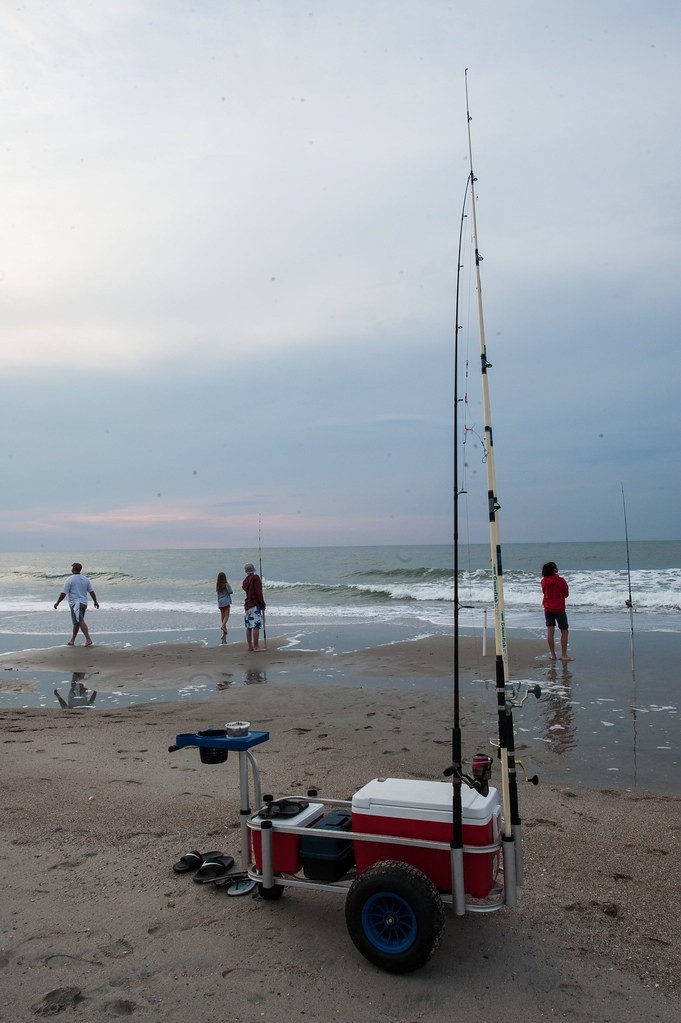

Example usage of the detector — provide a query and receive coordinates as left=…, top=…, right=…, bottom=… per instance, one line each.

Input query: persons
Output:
left=53, top=672, right=97, bottom=709
left=241, top=563, right=268, bottom=652
left=215, top=572, right=233, bottom=633
left=54, top=563, right=99, bottom=647
left=540, top=562, right=576, bottom=661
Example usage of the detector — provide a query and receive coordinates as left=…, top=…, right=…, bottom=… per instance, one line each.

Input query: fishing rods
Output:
left=258, top=513, right=269, bottom=649
left=442, top=174, right=475, bottom=914
left=463, top=66, right=526, bottom=886
left=627, top=610, right=638, bottom=784
left=620, top=480, right=633, bottom=609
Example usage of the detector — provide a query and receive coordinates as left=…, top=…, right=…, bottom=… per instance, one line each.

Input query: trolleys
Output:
left=164, top=725, right=520, bottom=972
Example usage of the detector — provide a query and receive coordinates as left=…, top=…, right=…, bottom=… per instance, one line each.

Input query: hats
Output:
left=243, top=564, right=255, bottom=574
left=72, top=562, right=83, bottom=571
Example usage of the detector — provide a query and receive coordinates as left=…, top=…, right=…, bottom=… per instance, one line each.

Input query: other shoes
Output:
left=560, top=656, right=574, bottom=661
left=549, top=655, right=556, bottom=660
